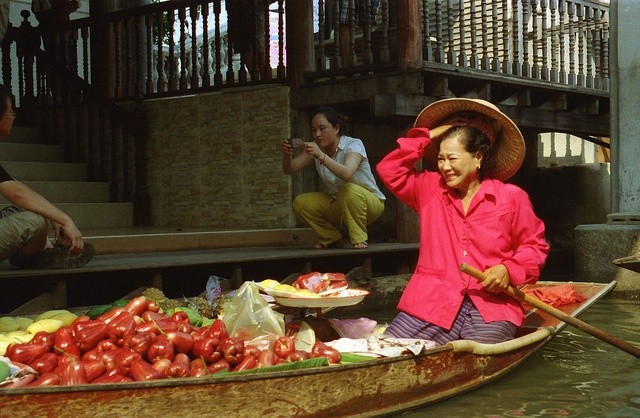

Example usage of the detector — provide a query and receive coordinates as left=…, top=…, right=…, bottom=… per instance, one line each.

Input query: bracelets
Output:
left=319, top=153, right=327, bottom=165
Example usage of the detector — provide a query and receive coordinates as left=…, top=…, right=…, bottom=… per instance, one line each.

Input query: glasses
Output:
left=2, top=111, right=16, bottom=119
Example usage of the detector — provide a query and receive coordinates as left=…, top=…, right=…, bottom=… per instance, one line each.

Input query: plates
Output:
left=264, top=286, right=372, bottom=307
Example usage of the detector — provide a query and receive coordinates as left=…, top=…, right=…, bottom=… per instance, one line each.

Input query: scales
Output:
left=273, top=288, right=370, bottom=344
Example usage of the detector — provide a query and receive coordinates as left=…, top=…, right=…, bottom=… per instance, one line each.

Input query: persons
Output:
left=281, top=105, right=386, bottom=249
left=1, top=84, right=85, bottom=264
left=376, top=98, right=552, bottom=347
left=331, top=1, right=379, bottom=70
left=225, top=0, right=276, bottom=81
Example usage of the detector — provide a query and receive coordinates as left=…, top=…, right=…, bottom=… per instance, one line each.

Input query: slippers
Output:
left=353, top=240, right=368, bottom=249
left=313, top=243, right=332, bottom=249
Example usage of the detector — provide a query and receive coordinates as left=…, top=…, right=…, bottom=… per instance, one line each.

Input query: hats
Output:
left=413, top=98, right=526, bottom=182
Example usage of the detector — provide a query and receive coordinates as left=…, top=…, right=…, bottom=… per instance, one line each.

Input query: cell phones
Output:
left=287, top=138, right=303, bottom=148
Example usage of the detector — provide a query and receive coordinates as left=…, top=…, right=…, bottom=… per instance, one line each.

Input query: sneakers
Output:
left=28, top=242, right=95, bottom=268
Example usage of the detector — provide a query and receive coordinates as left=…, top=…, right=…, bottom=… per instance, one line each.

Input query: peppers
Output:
left=292, top=271, right=350, bottom=296
left=6, top=295, right=342, bottom=389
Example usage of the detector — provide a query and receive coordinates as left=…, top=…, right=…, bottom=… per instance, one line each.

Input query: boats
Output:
left=0, top=272, right=616, bottom=418
